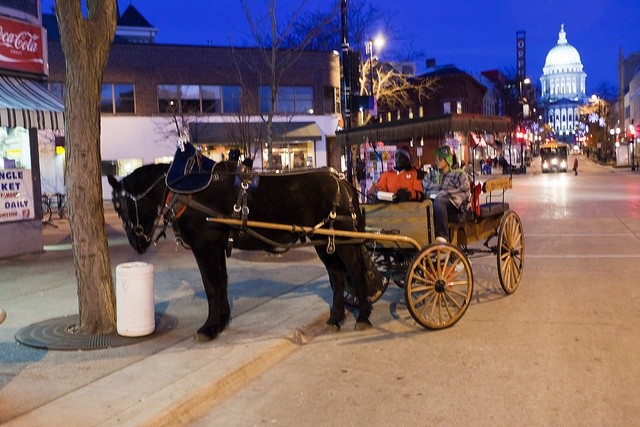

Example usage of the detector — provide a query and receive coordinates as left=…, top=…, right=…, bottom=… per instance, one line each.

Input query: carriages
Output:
left=106, top=115, right=524, bottom=343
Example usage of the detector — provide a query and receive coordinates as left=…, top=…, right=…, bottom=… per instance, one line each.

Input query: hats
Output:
left=395, top=146, right=411, bottom=161
left=435, top=146, right=453, bottom=165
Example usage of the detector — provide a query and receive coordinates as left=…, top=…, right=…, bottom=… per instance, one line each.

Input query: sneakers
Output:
left=436, top=236, right=448, bottom=244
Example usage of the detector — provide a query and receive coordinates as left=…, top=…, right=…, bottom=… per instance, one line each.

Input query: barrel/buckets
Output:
left=114, top=260, right=156, bottom=338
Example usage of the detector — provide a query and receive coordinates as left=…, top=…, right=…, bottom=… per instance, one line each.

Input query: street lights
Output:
left=629, top=118, right=637, bottom=171
left=609, top=127, right=621, bottom=145
left=518, top=77, right=532, bottom=115
left=367, top=32, right=385, bottom=99
left=592, top=94, right=601, bottom=120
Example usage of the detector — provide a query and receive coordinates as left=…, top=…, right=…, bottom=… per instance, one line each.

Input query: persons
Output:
left=461, top=159, right=466, bottom=176
left=369, top=145, right=423, bottom=204
left=298, top=152, right=304, bottom=168
left=295, top=155, right=299, bottom=169
left=308, top=160, right=313, bottom=169
left=452, top=149, right=459, bottom=169
left=355, top=154, right=367, bottom=205
left=480, top=160, right=490, bottom=174
left=470, top=173, right=481, bottom=223
left=487, top=156, right=492, bottom=168
left=573, top=157, right=579, bottom=174
left=501, top=157, right=508, bottom=170
left=499, top=153, right=503, bottom=163
left=468, top=162, right=473, bottom=177
left=243, top=158, right=253, bottom=170
left=284, top=164, right=288, bottom=173
left=228, top=149, right=240, bottom=162
left=421, top=144, right=471, bottom=245
left=494, top=156, right=498, bottom=167
left=270, top=156, right=282, bottom=170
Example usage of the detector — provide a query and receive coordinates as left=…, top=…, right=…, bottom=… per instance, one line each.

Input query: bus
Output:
left=539, top=140, right=570, bottom=172
left=501, top=143, right=527, bottom=175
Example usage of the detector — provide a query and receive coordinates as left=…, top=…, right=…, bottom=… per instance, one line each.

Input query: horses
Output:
left=105, top=160, right=376, bottom=345
